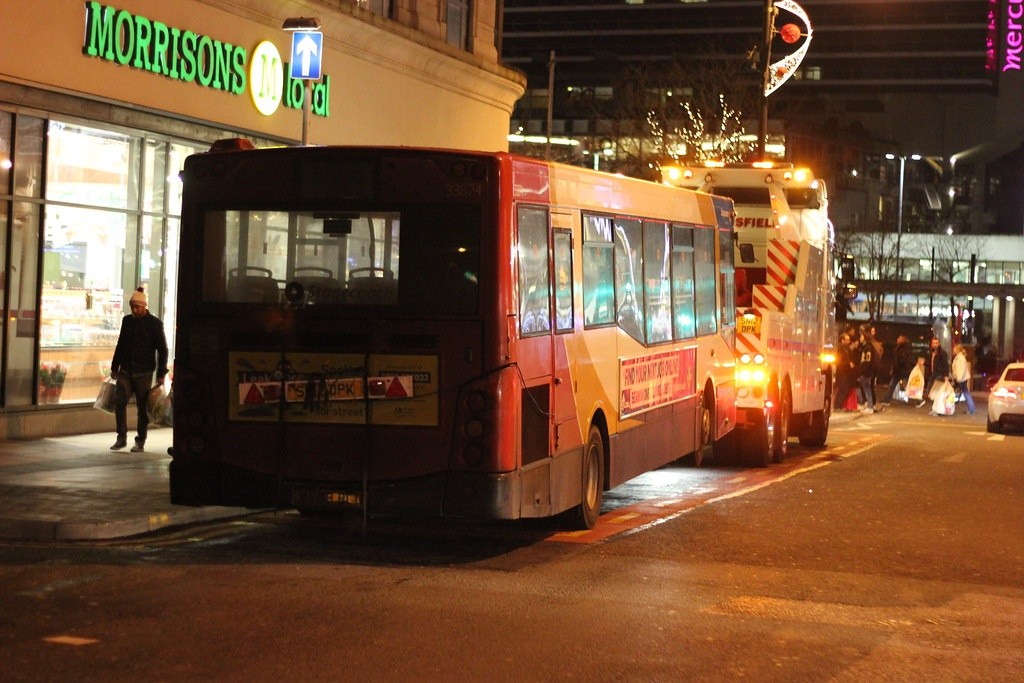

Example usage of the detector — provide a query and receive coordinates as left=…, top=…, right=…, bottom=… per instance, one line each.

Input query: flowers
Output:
left=40, top=363, right=67, bottom=389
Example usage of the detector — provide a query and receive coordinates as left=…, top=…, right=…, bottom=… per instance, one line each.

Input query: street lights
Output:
left=885, top=153, right=921, bottom=323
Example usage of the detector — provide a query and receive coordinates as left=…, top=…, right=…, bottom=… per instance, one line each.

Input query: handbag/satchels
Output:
left=93, top=380, right=116, bottom=416
left=147, top=383, right=174, bottom=427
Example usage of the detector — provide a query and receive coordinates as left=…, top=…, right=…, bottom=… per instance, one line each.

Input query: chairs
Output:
left=284, top=266, right=344, bottom=304
left=226, top=266, right=278, bottom=304
left=348, top=267, right=398, bottom=305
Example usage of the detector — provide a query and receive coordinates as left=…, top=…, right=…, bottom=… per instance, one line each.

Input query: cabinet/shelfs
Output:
left=39, top=287, right=125, bottom=382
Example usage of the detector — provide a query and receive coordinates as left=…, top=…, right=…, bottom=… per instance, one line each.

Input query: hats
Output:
left=129, top=286, right=149, bottom=307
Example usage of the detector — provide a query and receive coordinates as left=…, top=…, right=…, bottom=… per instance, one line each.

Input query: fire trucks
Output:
left=654, top=161, right=835, bottom=469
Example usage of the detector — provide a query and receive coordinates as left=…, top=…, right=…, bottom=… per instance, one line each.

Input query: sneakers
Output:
left=131, top=445, right=144, bottom=453
left=109, top=440, right=126, bottom=450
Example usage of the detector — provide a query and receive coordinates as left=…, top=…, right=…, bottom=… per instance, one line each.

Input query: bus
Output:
left=167, top=138, right=737, bottom=529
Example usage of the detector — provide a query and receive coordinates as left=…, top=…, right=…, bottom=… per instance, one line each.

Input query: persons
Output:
left=838, top=324, right=975, bottom=414
left=110, top=286, right=169, bottom=452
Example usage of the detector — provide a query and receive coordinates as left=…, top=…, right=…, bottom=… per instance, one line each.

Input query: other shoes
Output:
left=880, top=401, right=891, bottom=407
left=963, top=410, right=975, bottom=415
left=916, top=400, right=927, bottom=407
left=862, top=407, right=875, bottom=415
left=929, top=411, right=939, bottom=417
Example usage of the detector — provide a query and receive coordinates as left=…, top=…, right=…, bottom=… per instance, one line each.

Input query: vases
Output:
left=40, top=384, right=62, bottom=402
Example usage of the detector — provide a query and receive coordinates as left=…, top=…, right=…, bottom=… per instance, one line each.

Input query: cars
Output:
left=988, top=362, right=1024, bottom=432
